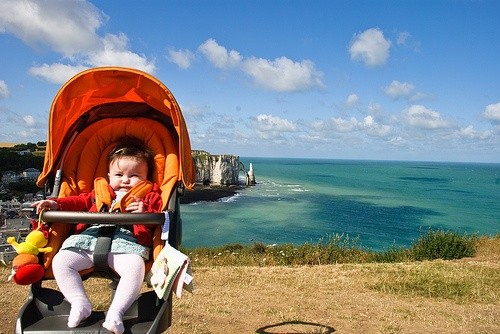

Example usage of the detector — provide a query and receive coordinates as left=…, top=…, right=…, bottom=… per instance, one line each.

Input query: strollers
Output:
left=15, top=67, right=196, bottom=333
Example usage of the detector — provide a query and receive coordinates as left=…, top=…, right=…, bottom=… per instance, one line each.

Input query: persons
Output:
left=31, top=143, right=163, bottom=334
